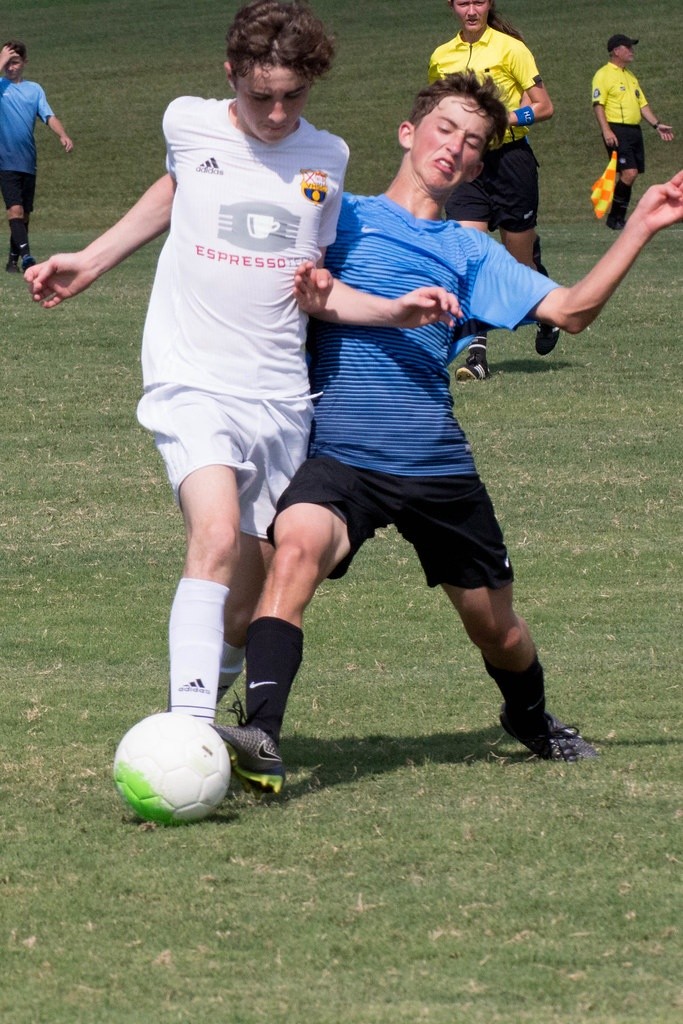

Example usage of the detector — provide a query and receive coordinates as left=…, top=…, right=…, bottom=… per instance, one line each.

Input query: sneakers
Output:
left=208, top=691, right=286, bottom=793
left=534, top=320, right=560, bottom=356
left=454, top=355, right=490, bottom=381
left=499, top=700, right=600, bottom=763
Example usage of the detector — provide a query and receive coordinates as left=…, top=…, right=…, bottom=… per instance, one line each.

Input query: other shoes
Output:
left=22, top=256, right=37, bottom=270
left=606, top=213, right=627, bottom=229
left=5, top=259, right=21, bottom=273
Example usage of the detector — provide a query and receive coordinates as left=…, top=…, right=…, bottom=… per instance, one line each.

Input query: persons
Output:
left=21, top=0, right=465, bottom=762
left=591, top=34, right=675, bottom=233
left=196, top=73, right=683, bottom=794
left=425, top=0, right=562, bottom=385
left=0, top=39, right=74, bottom=274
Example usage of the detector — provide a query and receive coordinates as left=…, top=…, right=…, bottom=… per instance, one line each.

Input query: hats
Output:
left=606, top=33, right=640, bottom=54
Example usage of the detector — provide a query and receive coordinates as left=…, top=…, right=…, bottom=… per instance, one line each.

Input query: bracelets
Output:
left=652, top=120, right=661, bottom=129
left=513, top=106, right=535, bottom=127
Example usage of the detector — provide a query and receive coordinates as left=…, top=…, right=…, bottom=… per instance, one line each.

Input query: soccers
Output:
left=106, top=708, right=239, bottom=830
